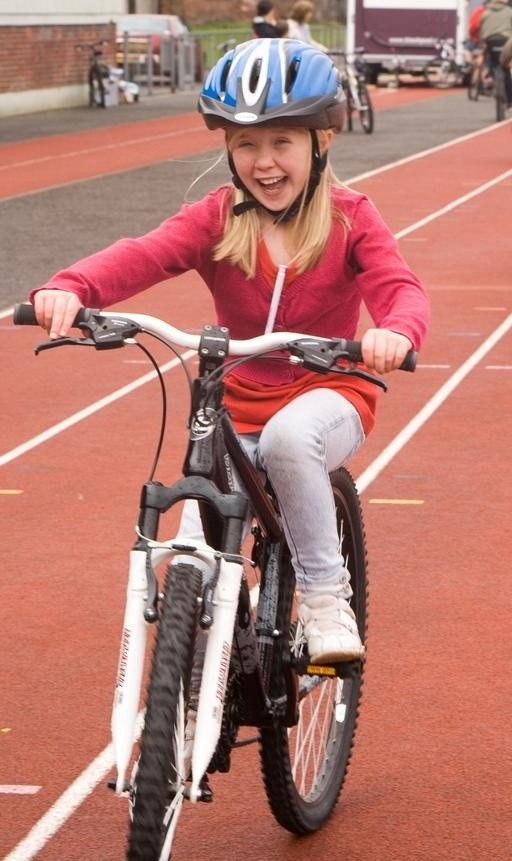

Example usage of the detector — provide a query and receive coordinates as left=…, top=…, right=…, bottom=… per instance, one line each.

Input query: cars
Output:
left=115, top=13, right=203, bottom=82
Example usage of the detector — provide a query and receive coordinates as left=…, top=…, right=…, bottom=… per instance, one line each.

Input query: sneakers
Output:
left=295, top=595, right=367, bottom=665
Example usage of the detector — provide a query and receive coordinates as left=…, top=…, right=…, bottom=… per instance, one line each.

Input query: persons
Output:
left=276, top=21, right=290, bottom=37
left=285, top=1, right=334, bottom=53
left=28, top=37, right=427, bottom=779
left=250, top=0, right=278, bottom=39
left=478, top=0, right=512, bottom=109
left=467, top=0, right=492, bottom=77
left=116, top=78, right=141, bottom=105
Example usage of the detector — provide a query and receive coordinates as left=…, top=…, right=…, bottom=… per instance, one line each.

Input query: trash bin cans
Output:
left=93, top=68, right=124, bottom=108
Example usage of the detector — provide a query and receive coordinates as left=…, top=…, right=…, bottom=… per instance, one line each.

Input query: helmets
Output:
left=197, top=37, right=347, bottom=133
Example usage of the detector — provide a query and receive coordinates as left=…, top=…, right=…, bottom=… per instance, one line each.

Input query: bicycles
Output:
left=334, top=47, right=374, bottom=136
left=13, top=304, right=418, bottom=861
left=424, top=44, right=511, bottom=122
left=72, top=39, right=112, bottom=109
